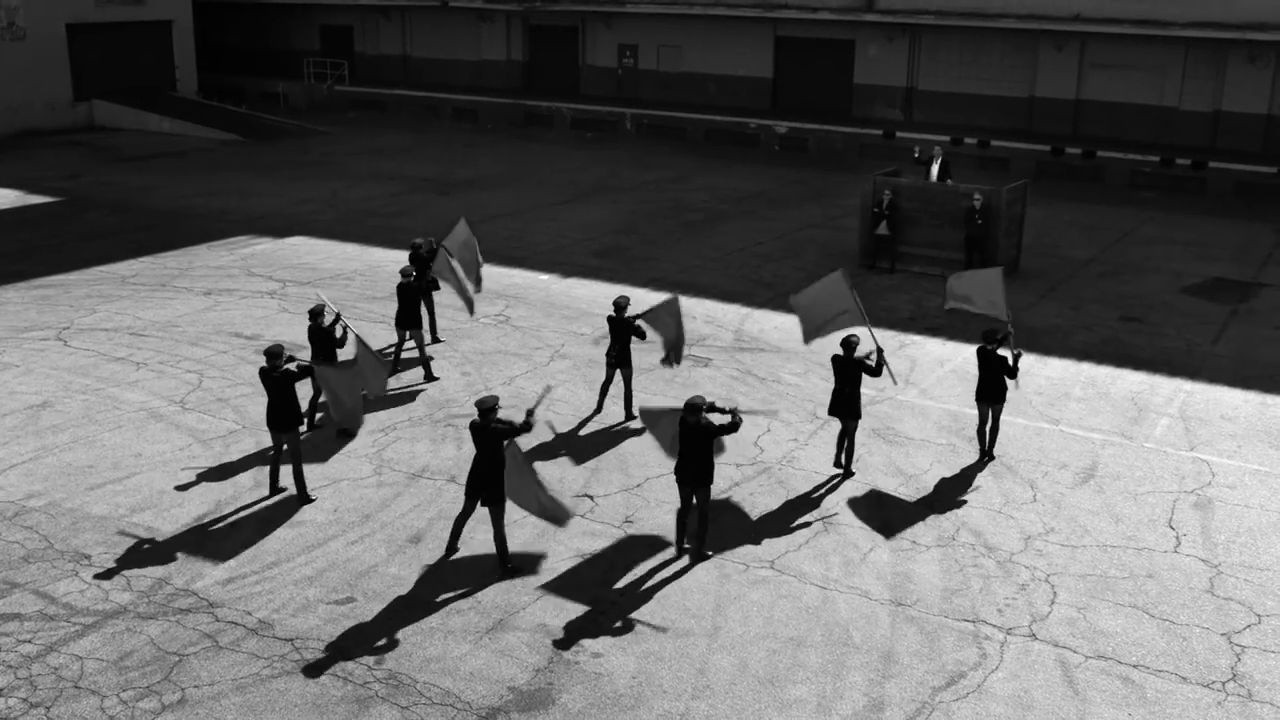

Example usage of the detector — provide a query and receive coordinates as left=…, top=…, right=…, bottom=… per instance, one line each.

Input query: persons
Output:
left=674, top=395, right=743, bottom=565
left=864, top=187, right=903, bottom=273
left=913, top=145, right=952, bottom=184
left=445, top=395, right=525, bottom=578
left=258, top=344, right=318, bottom=504
left=593, top=295, right=639, bottom=421
left=827, top=334, right=885, bottom=478
left=975, top=328, right=1023, bottom=462
left=392, top=237, right=446, bottom=381
left=960, top=190, right=992, bottom=270
left=306, top=304, right=357, bottom=437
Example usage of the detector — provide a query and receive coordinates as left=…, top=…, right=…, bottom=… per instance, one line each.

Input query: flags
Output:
left=639, top=296, right=685, bottom=368
left=312, top=335, right=392, bottom=427
left=504, top=439, right=571, bottom=527
left=944, top=267, right=1009, bottom=322
left=429, top=246, right=474, bottom=318
left=639, top=407, right=726, bottom=460
left=441, top=217, right=483, bottom=293
left=789, top=267, right=867, bottom=344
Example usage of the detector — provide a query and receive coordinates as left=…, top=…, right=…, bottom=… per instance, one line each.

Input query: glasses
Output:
left=972, top=198, right=981, bottom=201
left=881, top=193, right=891, bottom=196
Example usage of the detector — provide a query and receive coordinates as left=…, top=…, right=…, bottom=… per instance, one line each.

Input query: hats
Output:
left=399, top=265, right=415, bottom=276
left=982, top=328, right=1000, bottom=343
left=411, top=239, right=423, bottom=249
left=307, top=304, right=330, bottom=317
left=839, top=334, right=860, bottom=348
left=613, top=295, right=629, bottom=307
left=263, top=343, right=286, bottom=361
left=684, top=395, right=708, bottom=415
left=475, top=395, right=503, bottom=412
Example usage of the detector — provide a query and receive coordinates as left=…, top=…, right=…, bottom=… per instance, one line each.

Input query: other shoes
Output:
left=424, top=374, right=439, bottom=380
left=986, top=451, right=995, bottom=459
left=307, top=424, right=321, bottom=432
left=392, top=366, right=401, bottom=371
left=298, top=494, right=317, bottom=502
left=625, top=414, right=639, bottom=421
left=691, top=551, right=712, bottom=562
left=979, top=449, right=987, bottom=457
left=676, top=543, right=692, bottom=554
left=447, top=545, right=461, bottom=554
left=270, top=486, right=287, bottom=495
left=431, top=336, right=446, bottom=344
left=833, top=463, right=844, bottom=469
left=845, top=470, right=857, bottom=477
